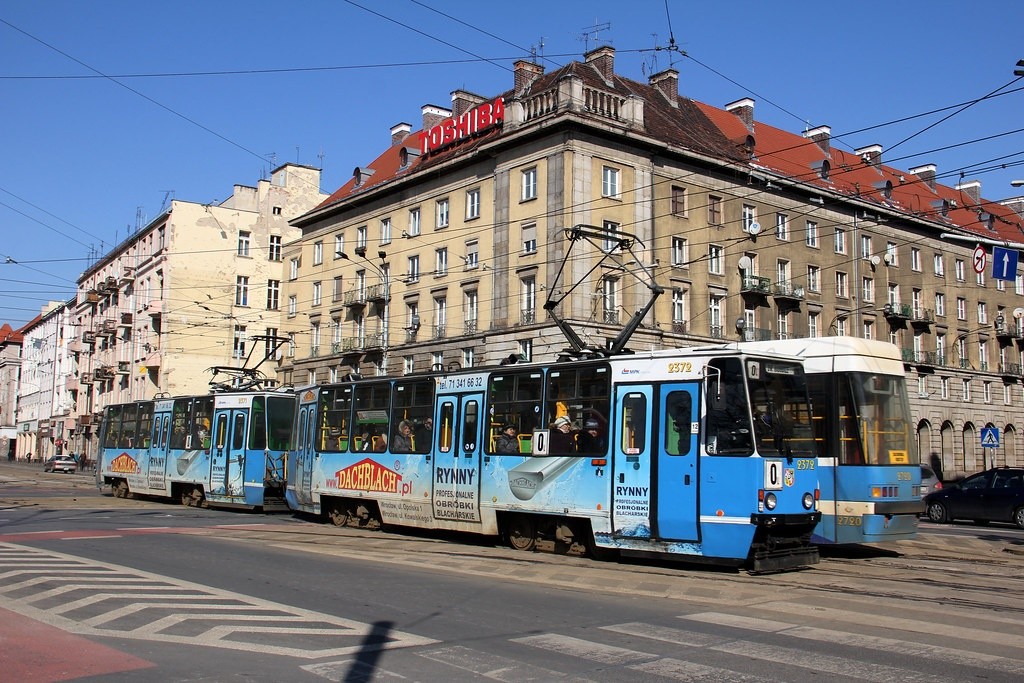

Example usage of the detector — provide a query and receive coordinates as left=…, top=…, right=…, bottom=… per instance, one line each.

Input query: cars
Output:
left=919, top=463, right=943, bottom=499
left=43, top=455, right=78, bottom=474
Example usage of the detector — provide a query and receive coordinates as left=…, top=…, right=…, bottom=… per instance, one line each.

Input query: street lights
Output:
left=221, top=314, right=242, bottom=387
left=9, top=377, right=41, bottom=438
left=334, top=246, right=388, bottom=377
left=827, top=304, right=886, bottom=337
left=950, top=325, right=1004, bottom=368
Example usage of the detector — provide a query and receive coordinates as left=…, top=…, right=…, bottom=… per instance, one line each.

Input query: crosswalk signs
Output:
left=980, top=428, right=999, bottom=447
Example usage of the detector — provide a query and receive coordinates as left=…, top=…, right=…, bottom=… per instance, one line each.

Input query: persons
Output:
left=326, top=427, right=341, bottom=450
left=359, top=423, right=388, bottom=451
left=74, top=452, right=79, bottom=462
left=175, top=425, right=189, bottom=448
left=122, top=430, right=134, bottom=447
left=69, top=451, right=74, bottom=458
left=139, top=431, right=150, bottom=447
left=107, top=432, right=117, bottom=447
left=549, top=417, right=576, bottom=453
left=26, top=453, right=32, bottom=464
left=495, top=422, right=519, bottom=453
left=78, top=451, right=86, bottom=471
left=192, top=424, right=208, bottom=449
left=577, top=419, right=601, bottom=454
left=394, top=420, right=414, bottom=451
left=8, top=450, right=13, bottom=462
left=415, top=416, right=432, bottom=451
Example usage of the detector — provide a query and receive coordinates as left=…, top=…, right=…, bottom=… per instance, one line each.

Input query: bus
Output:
left=95, top=335, right=297, bottom=514
left=284, top=221, right=924, bottom=577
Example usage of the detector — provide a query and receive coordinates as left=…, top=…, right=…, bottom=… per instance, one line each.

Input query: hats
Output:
left=583, top=420, right=601, bottom=429
left=501, top=421, right=519, bottom=433
left=554, top=416, right=571, bottom=428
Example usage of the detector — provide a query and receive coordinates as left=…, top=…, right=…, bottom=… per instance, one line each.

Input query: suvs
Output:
left=921, top=465, right=1024, bottom=530
